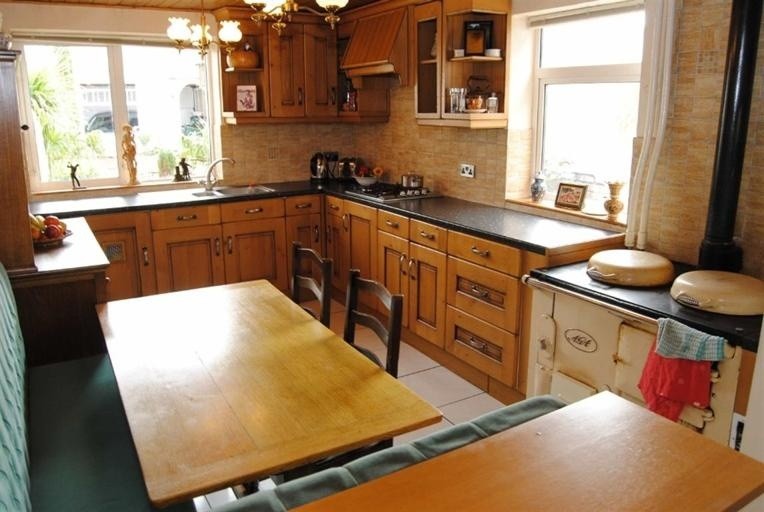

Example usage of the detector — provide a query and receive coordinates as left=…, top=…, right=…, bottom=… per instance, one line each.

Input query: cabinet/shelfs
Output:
left=0, top=48, right=112, bottom=365
left=267, top=13, right=339, bottom=123
left=523, top=248, right=764, bottom=447
left=337, top=0, right=391, bottom=123
left=29, top=180, right=627, bottom=406
left=409, top=0, right=512, bottom=130
left=217, top=0, right=268, bottom=125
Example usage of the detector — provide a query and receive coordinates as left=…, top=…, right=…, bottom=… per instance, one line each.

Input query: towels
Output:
left=655, top=317, right=726, bottom=363
left=638, top=338, right=711, bottom=421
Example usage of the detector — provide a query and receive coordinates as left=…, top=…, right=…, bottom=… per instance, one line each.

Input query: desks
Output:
left=96, top=279, right=446, bottom=507
left=285, top=389, right=764, bottom=512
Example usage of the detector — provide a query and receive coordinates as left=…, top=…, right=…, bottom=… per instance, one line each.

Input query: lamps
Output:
left=165, top=16, right=241, bottom=58
left=244, top=0, right=350, bottom=36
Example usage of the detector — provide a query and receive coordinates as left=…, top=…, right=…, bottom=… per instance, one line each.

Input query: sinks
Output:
left=216, top=185, right=276, bottom=198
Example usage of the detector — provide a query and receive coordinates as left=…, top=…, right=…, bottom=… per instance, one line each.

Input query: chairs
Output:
left=275, top=267, right=404, bottom=485
left=291, top=241, right=332, bottom=326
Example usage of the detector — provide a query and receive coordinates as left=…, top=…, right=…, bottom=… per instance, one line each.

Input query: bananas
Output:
left=30, top=212, right=47, bottom=232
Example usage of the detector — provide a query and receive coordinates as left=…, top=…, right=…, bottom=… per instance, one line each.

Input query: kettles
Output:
left=310, top=152, right=328, bottom=182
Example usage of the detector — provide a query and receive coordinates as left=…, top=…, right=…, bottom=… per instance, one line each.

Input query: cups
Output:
left=450, top=88, right=466, bottom=113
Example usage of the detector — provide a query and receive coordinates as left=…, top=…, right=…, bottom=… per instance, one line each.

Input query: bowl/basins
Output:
left=352, top=176, right=377, bottom=187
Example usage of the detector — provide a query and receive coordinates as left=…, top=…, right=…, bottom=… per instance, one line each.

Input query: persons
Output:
left=117, top=123, right=143, bottom=185
left=66, top=161, right=82, bottom=187
left=179, top=157, right=191, bottom=179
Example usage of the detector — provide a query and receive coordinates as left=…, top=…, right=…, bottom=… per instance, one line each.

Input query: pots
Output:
left=670, top=270, right=764, bottom=316
left=587, top=249, right=674, bottom=288
left=402, top=170, right=423, bottom=188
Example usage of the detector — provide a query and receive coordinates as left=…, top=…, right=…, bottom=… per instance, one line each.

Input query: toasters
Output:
left=338, top=158, right=357, bottom=180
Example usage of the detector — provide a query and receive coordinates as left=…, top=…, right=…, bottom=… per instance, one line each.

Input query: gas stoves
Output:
left=344, top=184, right=443, bottom=204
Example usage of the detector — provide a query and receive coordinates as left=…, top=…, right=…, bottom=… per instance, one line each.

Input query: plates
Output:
left=32, top=230, right=72, bottom=244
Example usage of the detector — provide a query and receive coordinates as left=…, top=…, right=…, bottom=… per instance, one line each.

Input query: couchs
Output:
left=0, top=263, right=198, bottom=512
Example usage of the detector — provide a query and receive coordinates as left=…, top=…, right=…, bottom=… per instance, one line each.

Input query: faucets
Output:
left=199, top=157, right=235, bottom=191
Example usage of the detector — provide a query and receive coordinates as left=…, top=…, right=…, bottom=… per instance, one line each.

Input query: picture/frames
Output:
left=236, top=85, right=257, bottom=112
